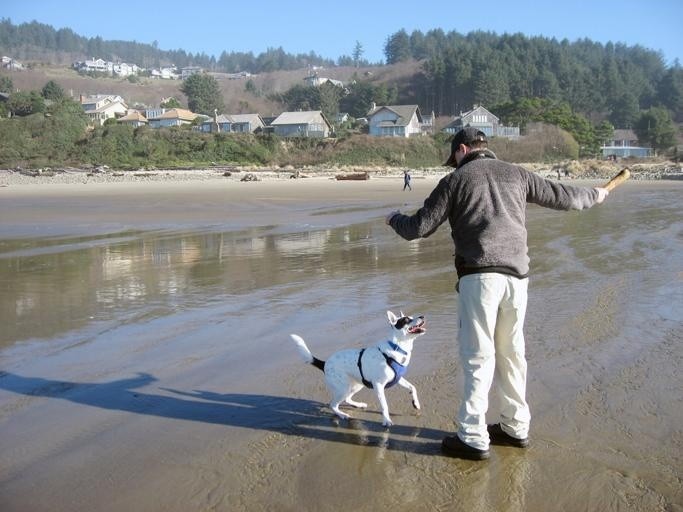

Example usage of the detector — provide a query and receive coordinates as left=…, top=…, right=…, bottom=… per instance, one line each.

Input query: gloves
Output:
left=594, top=187, right=609, bottom=205
left=384, top=209, right=400, bottom=225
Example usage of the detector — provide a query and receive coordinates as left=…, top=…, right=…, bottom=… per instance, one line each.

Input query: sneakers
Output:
left=439, top=434, right=491, bottom=461
left=486, top=420, right=529, bottom=449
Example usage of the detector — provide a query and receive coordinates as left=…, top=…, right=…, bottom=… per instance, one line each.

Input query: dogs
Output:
left=289, top=310, right=427, bottom=428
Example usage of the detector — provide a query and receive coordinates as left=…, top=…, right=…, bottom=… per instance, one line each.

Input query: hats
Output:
left=439, top=126, right=487, bottom=167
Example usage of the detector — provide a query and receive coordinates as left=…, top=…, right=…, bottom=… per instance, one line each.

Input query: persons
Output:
left=402, top=170, right=411, bottom=192
left=383, top=124, right=611, bottom=462
